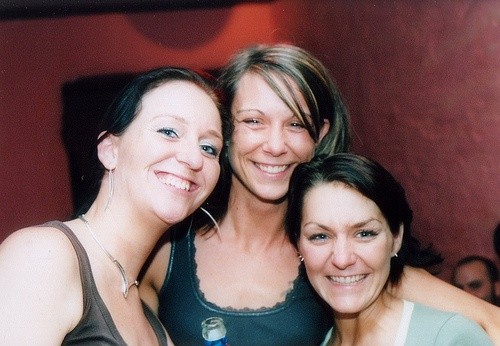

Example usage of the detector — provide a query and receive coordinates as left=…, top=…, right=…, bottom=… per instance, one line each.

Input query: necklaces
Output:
left=79, top=214, right=139, bottom=298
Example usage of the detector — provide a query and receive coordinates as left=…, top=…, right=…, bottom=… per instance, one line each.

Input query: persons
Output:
left=0, top=68, right=227, bottom=346
left=140, top=42, right=500, bottom=346
left=287, top=153, right=500, bottom=345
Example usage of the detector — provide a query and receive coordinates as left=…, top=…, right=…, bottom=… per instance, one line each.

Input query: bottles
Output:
left=201, top=317, right=229, bottom=346
